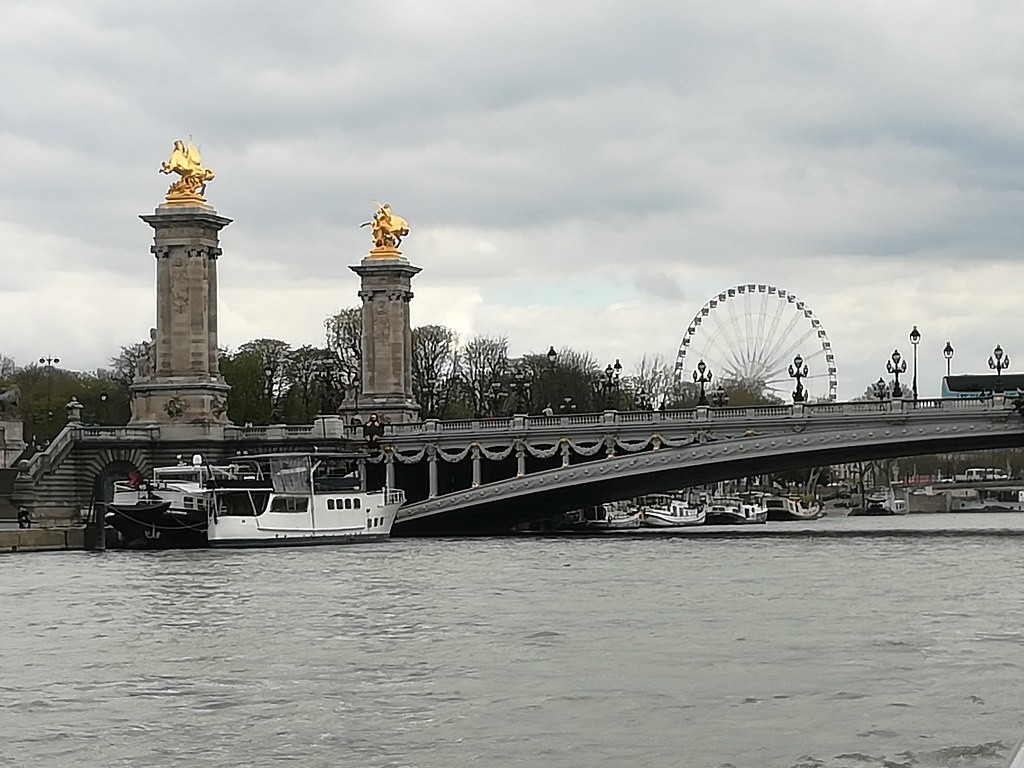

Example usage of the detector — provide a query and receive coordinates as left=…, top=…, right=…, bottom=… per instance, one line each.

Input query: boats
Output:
left=102, top=451, right=407, bottom=550
left=951, top=486, right=1024, bottom=513
left=563, top=491, right=821, bottom=531
left=864, top=494, right=907, bottom=516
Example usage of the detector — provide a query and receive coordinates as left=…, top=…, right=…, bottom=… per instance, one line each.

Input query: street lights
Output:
left=788, top=353, right=809, bottom=402
left=39, top=353, right=60, bottom=411
left=712, top=385, right=729, bottom=406
left=988, top=345, right=1010, bottom=376
left=910, top=326, right=922, bottom=409
left=943, top=341, right=954, bottom=376
left=886, top=349, right=906, bottom=398
left=692, top=359, right=714, bottom=406
left=604, top=363, right=613, bottom=408
left=873, top=377, right=890, bottom=401
left=614, top=358, right=623, bottom=412
left=546, top=344, right=559, bottom=413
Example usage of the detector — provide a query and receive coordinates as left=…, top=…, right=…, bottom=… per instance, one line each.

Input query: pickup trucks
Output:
left=954, top=468, right=1007, bottom=482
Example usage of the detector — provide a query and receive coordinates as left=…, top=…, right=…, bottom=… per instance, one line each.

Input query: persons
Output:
left=344, top=412, right=383, bottom=442
left=17, top=506, right=31, bottom=529
left=469, top=400, right=666, bottom=424
left=420, top=409, right=446, bottom=432
